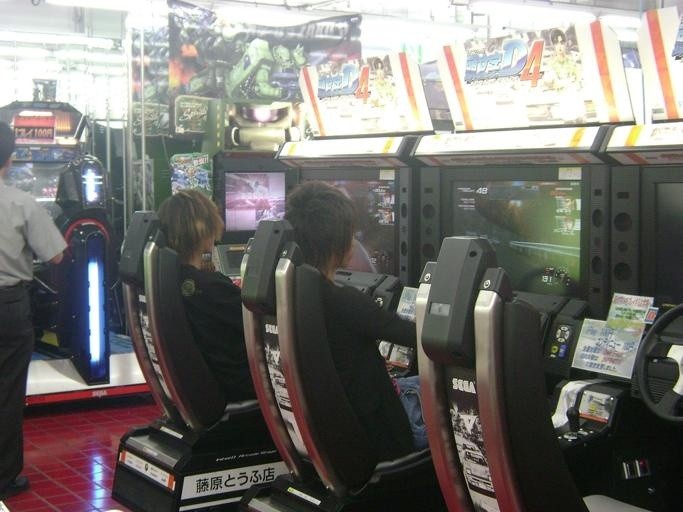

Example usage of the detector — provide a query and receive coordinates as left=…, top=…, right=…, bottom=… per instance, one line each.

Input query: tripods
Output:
left=226, top=172, right=286, bottom=231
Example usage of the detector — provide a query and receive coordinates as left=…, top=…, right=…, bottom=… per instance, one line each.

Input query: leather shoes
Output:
left=0, top=475, right=29, bottom=498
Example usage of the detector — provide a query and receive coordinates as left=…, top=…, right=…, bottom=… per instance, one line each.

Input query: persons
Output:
left=544, top=30, right=583, bottom=90
left=155, top=189, right=258, bottom=398
left=286, top=180, right=429, bottom=463
left=371, top=60, right=392, bottom=106
left=0, top=122, right=68, bottom=500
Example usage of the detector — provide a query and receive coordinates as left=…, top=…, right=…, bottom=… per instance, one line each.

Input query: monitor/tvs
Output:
left=300, top=179, right=395, bottom=275
left=656, top=183, right=683, bottom=306
left=453, top=180, right=581, bottom=297
left=2, top=161, right=67, bottom=201
